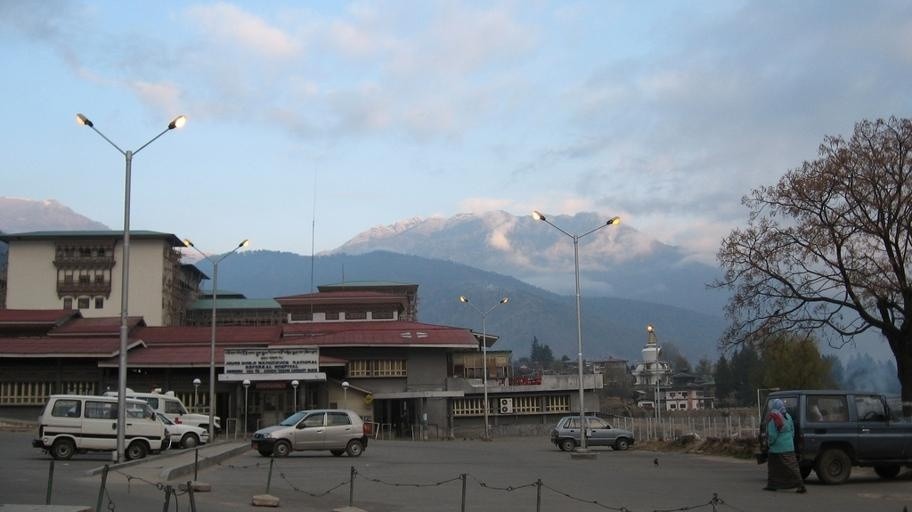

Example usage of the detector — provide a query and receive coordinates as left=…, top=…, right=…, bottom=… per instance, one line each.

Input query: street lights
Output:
left=242, top=378, right=251, bottom=434
left=648, top=326, right=663, bottom=441
left=76, top=111, right=187, bottom=461
left=533, top=209, right=620, bottom=448
left=291, top=380, right=299, bottom=414
left=461, top=296, right=510, bottom=439
left=757, top=387, right=781, bottom=425
left=185, top=238, right=250, bottom=438
left=192, top=378, right=201, bottom=407
left=342, top=382, right=349, bottom=409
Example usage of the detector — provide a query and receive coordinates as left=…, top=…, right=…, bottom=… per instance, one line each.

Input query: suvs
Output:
left=104, top=391, right=222, bottom=442
left=757, top=391, right=912, bottom=486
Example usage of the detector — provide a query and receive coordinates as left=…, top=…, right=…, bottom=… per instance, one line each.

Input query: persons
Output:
left=393, top=410, right=407, bottom=438
left=764, top=397, right=808, bottom=493
left=807, top=397, right=824, bottom=421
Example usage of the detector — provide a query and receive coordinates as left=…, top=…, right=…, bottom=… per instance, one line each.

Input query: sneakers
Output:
left=797, top=487, right=807, bottom=493
left=763, top=487, right=777, bottom=491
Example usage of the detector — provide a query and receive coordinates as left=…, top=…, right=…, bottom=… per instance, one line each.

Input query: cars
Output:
left=156, top=413, right=209, bottom=450
left=249, top=409, right=367, bottom=459
left=551, top=414, right=635, bottom=451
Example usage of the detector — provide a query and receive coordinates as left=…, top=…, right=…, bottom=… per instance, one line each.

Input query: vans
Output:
left=33, top=395, right=171, bottom=460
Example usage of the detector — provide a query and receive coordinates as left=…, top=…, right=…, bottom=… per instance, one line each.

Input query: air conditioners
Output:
left=500, top=398, right=512, bottom=414
